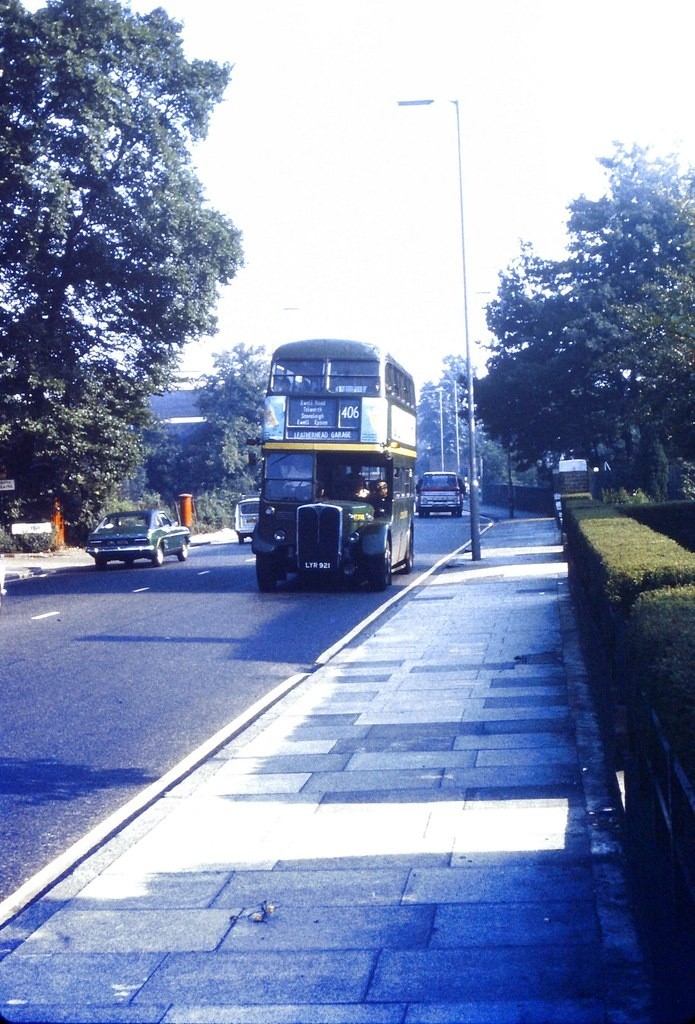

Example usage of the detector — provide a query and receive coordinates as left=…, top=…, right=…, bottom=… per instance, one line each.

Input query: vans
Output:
left=235, top=494, right=259, bottom=543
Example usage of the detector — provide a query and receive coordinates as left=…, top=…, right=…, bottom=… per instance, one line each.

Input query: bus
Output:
left=250, top=338, right=419, bottom=593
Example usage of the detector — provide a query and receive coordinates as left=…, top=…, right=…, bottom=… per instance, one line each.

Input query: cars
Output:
left=85, top=510, right=191, bottom=571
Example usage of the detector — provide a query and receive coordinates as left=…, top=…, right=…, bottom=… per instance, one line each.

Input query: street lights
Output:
left=399, top=95, right=485, bottom=561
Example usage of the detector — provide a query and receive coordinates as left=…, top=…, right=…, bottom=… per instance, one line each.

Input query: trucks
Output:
left=419, top=472, right=466, bottom=518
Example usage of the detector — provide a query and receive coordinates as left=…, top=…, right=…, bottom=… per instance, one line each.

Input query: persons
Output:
left=279, top=378, right=291, bottom=394
